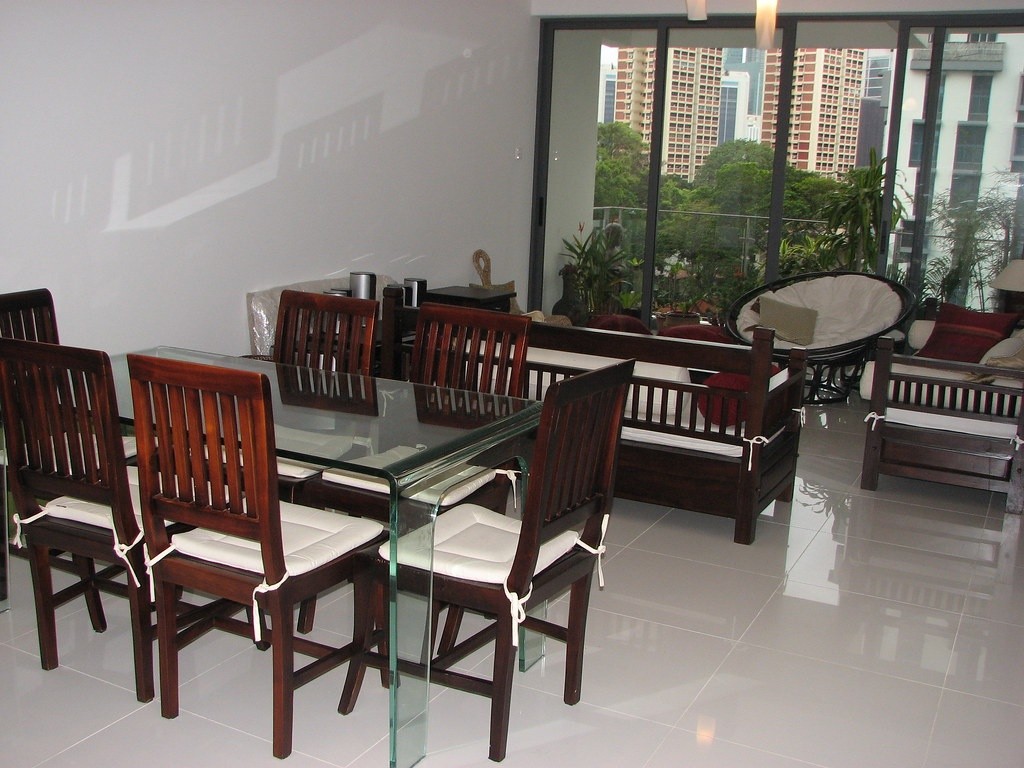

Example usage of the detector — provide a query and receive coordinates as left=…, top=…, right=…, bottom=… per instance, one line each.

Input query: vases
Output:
left=552, top=276, right=587, bottom=326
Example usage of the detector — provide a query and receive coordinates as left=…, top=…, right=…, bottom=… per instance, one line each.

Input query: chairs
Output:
left=726, top=272, right=916, bottom=404
left=0, top=288, right=635, bottom=762
left=469, top=250, right=572, bottom=327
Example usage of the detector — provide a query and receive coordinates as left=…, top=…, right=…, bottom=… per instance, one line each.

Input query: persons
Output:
left=603, top=223, right=625, bottom=268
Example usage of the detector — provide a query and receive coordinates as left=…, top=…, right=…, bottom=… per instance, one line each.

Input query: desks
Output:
left=26, top=346, right=545, bottom=768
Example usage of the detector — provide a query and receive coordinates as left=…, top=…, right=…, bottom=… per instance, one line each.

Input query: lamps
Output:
left=989, top=259, right=1024, bottom=313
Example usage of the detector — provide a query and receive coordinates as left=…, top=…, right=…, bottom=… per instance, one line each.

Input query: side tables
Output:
left=428, top=286, right=517, bottom=341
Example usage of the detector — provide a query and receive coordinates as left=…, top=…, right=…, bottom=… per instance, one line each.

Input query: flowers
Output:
left=559, top=265, right=576, bottom=276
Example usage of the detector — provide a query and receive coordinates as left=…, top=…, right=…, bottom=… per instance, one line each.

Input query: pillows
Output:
left=915, top=303, right=1024, bottom=364
left=587, top=315, right=653, bottom=335
left=699, top=363, right=781, bottom=426
left=745, top=296, right=817, bottom=345
left=658, top=325, right=736, bottom=345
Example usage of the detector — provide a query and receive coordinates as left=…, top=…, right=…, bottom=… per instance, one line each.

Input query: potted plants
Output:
left=561, top=221, right=720, bottom=331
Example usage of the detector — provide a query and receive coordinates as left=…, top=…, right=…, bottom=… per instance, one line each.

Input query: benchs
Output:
left=382, top=284, right=807, bottom=545
left=861, top=298, right=1024, bottom=514
